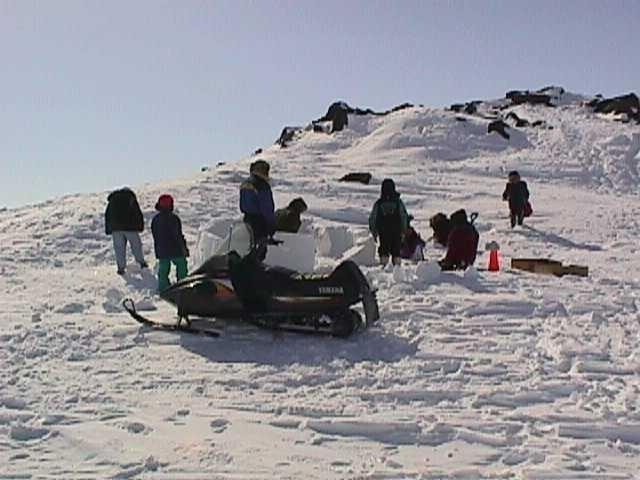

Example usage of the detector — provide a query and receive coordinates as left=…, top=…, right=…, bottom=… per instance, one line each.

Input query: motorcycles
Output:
left=122, top=238, right=379, bottom=338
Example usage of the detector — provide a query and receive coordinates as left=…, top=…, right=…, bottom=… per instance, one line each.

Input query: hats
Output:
left=159, top=195, right=173, bottom=208
left=250, top=161, right=270, bottom=177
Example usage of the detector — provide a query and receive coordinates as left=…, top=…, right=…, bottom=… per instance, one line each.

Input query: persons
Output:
left=151, top=194, right=191, bottom=290
left=503, top=170, right=531, bottom=230
left=368, top=178, right=409, bottom=267
left=439, top=209, right=480, bottom=272
left=240, top=158, right=276, bottom=262
left=104, top=186, right=149, bottom=274
left=271, top=196, right=308, bottom=234
